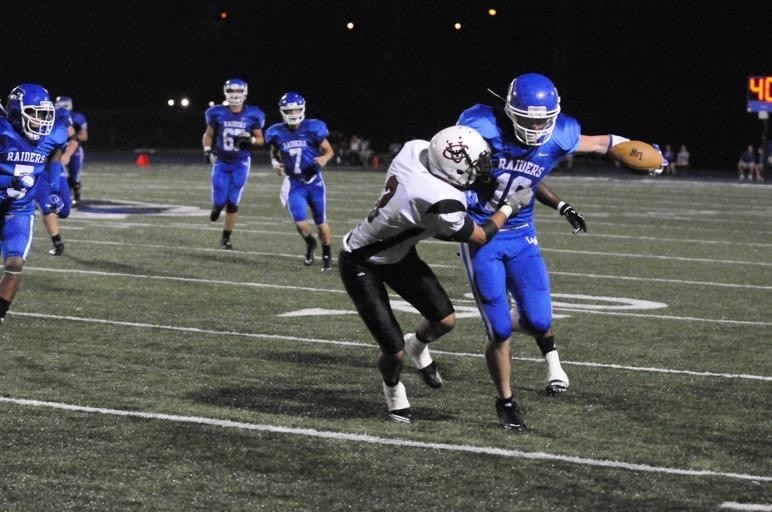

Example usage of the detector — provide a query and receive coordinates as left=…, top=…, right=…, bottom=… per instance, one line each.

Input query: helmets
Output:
left=427, top=125, right=494, bottom=190
left=7, top=83, right=56, bottom=140
left=505, top=73, right=561, bottom=147
left=55, top=95, right=73, bottom=117
left=279, top=92, right=306, bottom=125
left=223, top=78, right=248, bottom=107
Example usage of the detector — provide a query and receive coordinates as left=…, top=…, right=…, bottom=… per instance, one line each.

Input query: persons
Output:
left=329, top=132, right=405, bottom=171
left=335, top=125, right=535, bottom=425
left=453, top=71, right=669, bottom=433
left=736, top=145, right=770, bottom=181
left=52, top=91, right=90, bottom=210
left=263, top=90, right=335, bottom=271
left=531, top=179, right=589, bottom=398
left=566, top=140, right=688, bottom=178
left=0, top=84, right=72, bottom=334
left=34, top=105, right=80, bottom=256
left=200, top=76, right=265, bottom=250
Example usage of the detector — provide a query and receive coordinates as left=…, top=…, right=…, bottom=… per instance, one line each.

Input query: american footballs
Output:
left=608, top=140, right=661, bottom=171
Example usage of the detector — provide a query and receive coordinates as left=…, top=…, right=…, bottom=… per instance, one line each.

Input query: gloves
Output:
left=560, top=202, right=587, bottom=235
left=505, top=188, right=533, bottom=214
left=204, top=150, right=215, bottom=164
left=16, top=174, right=35, bottom=189
left=271, top=158, right=285, bottom=177
left=649, top=143, right=669, bottom=177
left=234, top=132, right=254, bottom=148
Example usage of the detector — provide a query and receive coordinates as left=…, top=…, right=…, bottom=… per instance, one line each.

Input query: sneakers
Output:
left=49, top=242, right=64, bottom=255
left=320, top=253, right=332, bottom=272
left=545, top=369, right=570, bottom=395
left=305, top=242, right=317, bottom=265
left=222, top=239, right=232, bottom=249
left=383, top=380, right=413, bottom=423
left=210, top=205, right=222, bottom=221
left=404, top=332, right=443, bottom=387
left=495, top=395, right=528, bottom=433
